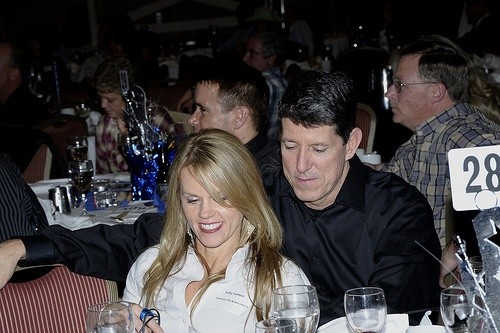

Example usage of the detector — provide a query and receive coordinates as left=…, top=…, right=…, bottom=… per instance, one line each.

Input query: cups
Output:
left=440, top=287, right=476, bottom=333
left=68, top=134, right=88, bottom=161
left=48, top=185, right=71, bottom=215
left=86, top=301, right=135, bottom=333
left=358, top=154, right=381, bottom=165
left=270, top=284, right=320, bottom=333
left=93, top=178, right=111, bottom=210
left=343, top=287, right=387, bottom=333
left=254, top=317, right=297, bottom=333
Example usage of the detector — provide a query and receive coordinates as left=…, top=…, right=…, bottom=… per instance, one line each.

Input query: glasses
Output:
left=246, top=48, right=266, bottom=59
left=387, top=78, right=440, bottom=93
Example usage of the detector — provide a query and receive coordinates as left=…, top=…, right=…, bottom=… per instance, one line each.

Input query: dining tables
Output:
left=26, top=171, right=158, bottom=232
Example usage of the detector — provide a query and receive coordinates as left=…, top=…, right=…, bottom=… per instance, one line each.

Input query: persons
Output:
left=1, top=154, right=54, bottom=283
left=455, top=1, right=499, bottom=124
left=381, top=34, right=499, bottom=332
left=188, top=68, right=280, bottom=184
left=2, top=12, right=291, bottom=173
left=122, top=128, right=312, bottom=333
left=0, top=72, right=442, bottom=329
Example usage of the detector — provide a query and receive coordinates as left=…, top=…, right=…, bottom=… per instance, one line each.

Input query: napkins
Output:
left=315, top=312, right=409, bottom=333
left=54, top=210, right=90, bottom=231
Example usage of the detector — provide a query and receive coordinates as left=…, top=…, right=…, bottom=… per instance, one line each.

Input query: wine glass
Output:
left=69, top=160, right=96, bottom=217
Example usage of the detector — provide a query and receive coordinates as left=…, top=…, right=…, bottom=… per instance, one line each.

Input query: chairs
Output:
left=32, top=114, right=89, bottom=145
left=0, top=263, right=119, bottom=333
left=23, top=143, right=53, bottom=183
left=127, top=85, right=146, bottom=123
left=353, top=102, right=377, bottom=155
left=333, top=46, right=390, bottom=104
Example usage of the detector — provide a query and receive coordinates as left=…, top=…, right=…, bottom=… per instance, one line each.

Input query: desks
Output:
left=324, top=35, right=347, bottom=60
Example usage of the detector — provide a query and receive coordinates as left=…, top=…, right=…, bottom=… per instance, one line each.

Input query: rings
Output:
left=139, top=307, right=154, bottom=324
